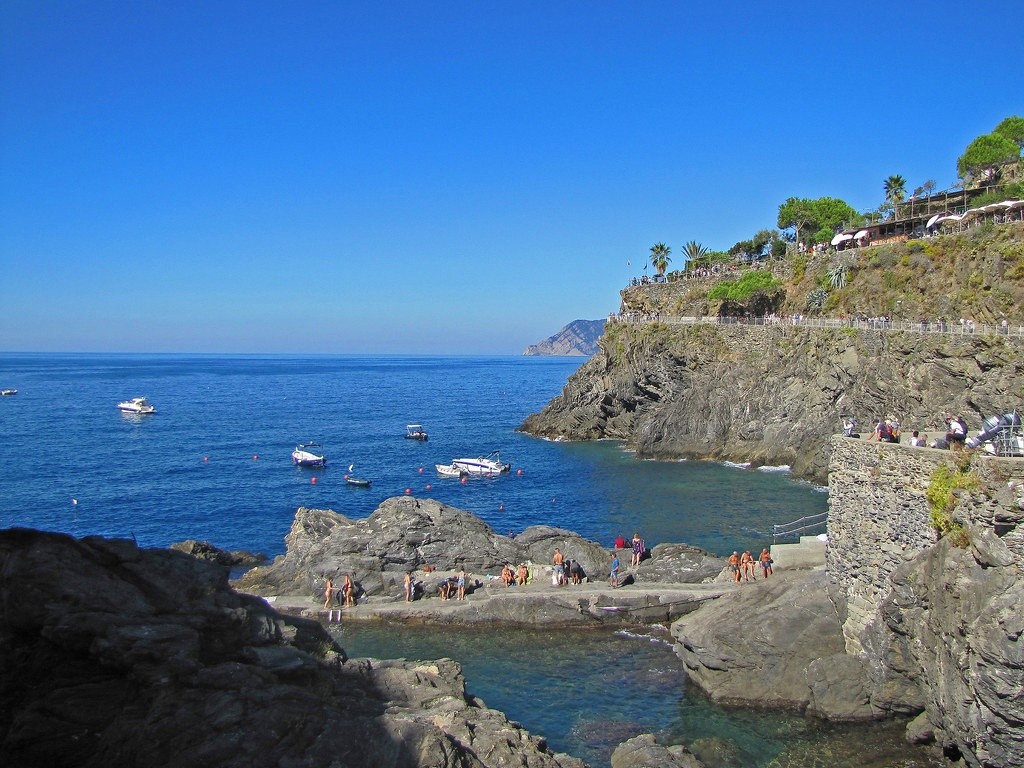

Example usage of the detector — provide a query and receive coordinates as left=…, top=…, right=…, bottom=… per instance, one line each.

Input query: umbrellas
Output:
left=926, top=214, right=939, bottom=228
left=830, top=229, right=868, bottom=245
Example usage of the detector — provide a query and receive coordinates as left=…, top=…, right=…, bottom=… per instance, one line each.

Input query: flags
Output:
left=627, top=260, right=631, bottom=266
left=643, top=264, right=647, bottom=269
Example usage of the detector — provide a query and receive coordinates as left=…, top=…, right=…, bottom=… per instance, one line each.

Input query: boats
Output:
left=405, top=425, right=428, bottom=440
left=292, top=441, right=328, bottom=467
left=966, top=412, right=1024, bottom=458
left=118, top=397, right=155, bottom=414
left=436, top=464, right=470, bottom=477
left=452, top=450, right=512, bottom=474
left=2, top=389, right=18, bottom=396
left=348, top=477, right=371, bottom=486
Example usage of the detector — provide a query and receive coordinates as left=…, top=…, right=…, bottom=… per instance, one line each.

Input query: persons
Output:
left=437, top=566, right=467, bottom=601
left=866, top=417, right=901, bottom=443
left=552, top=548, right=580, bottom=585
left=759, top=548, right=773, bottom=578
left=945, top=413, right=968, bottom=442
left=615, top=533, right=642, bottom=566
left=404, top=572, right=414, bottom=603
left=502, top=563, right=528, bottom=587
left=609, top=554, right=619, bottom=588
left=741, top=551, right=756, bottom=580
left=727, top=551, right=740, bottom=582
left=842, top=417, right=855, bottom=435
left=911, top=431, right=927, bottom=446
left=324, top=574, right=353, bottom=609
left=696, top=264, right=730, bottom=277
left=798, top=240, right=829, bottom=250
left=632, top=275, right=648, bottom=285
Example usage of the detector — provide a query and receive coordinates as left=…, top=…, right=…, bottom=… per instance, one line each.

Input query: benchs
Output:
left=511, top=571, right=532, bottom=584
